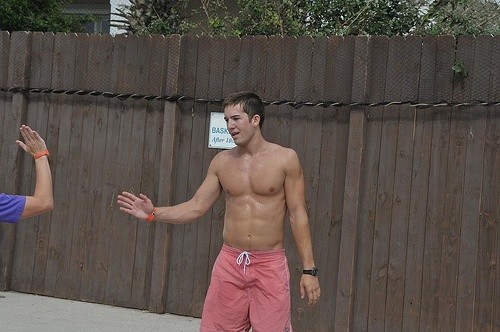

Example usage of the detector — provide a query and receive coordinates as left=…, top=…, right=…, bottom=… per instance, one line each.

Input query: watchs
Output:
left=303, top=266, right=321, bottom=277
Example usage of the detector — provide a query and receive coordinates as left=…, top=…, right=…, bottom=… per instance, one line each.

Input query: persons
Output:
left=0, top=124, right=54, bottom=224
left=117, top=92, right=321, bottom=332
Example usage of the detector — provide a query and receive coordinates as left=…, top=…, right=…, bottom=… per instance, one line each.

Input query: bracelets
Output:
left=35, top=148, right=50, bottom=158
left=146, top=207, right=157, bottom=222
